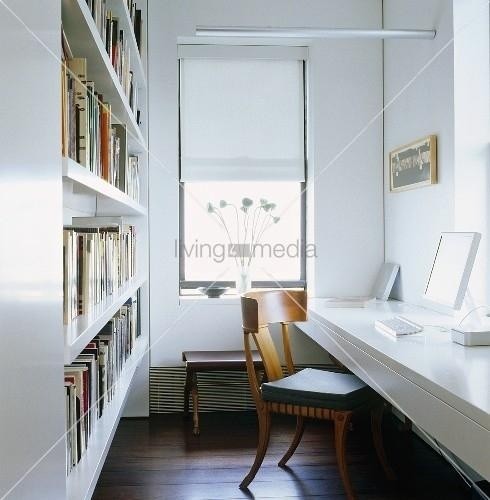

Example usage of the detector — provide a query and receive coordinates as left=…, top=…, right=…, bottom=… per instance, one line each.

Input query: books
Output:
left=369, top=262, right=400, bottom=301
left=61, top=0, right=143, bottom=483
left=326, top=296, right=377, bottom=308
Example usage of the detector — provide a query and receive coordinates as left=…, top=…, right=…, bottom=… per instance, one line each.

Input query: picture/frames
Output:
left=388, top=135, right=438, bottom=193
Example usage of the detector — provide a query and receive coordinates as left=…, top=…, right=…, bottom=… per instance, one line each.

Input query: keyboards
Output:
left=375, top=314, right=424, bottom=337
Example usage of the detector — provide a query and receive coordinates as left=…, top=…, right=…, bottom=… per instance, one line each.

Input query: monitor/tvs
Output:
left=424, top=230, right=483, bottom=331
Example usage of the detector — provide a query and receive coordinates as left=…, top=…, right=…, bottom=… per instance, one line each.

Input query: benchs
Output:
left=178, top=349, right=265, bottom=437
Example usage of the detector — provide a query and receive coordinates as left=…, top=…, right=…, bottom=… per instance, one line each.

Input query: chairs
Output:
left=236, top=288, right=395, bottom=499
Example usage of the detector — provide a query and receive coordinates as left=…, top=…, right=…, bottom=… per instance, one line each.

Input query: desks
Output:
left=293, top=294, right=490, bottom=499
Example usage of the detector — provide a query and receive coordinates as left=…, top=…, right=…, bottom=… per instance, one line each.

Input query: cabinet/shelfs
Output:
left=0, top=0, right=153, bottom=500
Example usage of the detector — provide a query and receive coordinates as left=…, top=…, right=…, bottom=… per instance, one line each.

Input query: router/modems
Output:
left=451, top=326, right=490, bottom=347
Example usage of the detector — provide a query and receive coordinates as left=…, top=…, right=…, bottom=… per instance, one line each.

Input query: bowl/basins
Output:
left=197, top=287, right=230, bottom=298
